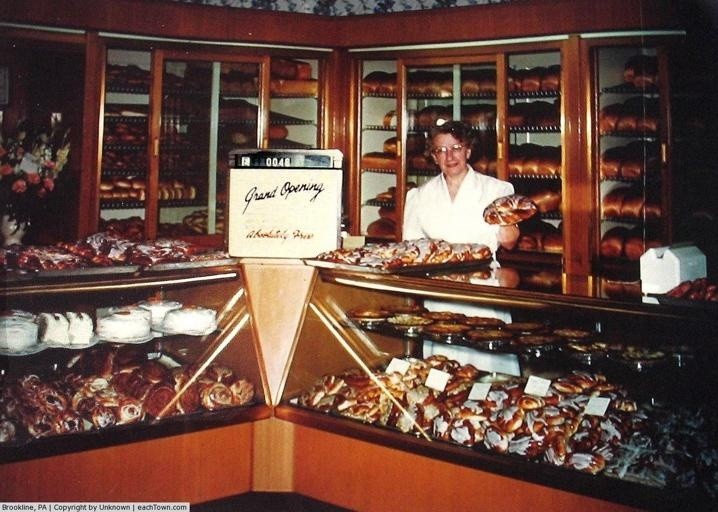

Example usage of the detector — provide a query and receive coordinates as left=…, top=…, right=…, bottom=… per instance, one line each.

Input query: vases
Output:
left=0, top=203, right=34, bottom=251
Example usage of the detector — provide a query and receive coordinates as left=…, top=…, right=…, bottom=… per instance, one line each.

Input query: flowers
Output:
left=1, top=102, right=78, bottom=239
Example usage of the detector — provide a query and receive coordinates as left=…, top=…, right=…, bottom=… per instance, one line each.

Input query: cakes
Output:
left=1, top=296, right=218, bottom=353
left=344, top=304, right=716, bottom=378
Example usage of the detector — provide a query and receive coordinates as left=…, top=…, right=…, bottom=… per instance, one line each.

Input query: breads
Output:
left=301, top=354, right=717, bottom=496
left=320, top=56, right=718, bottom=301
left=1, top=360, right=255, bottom=445
left=1, top=40, right=317, bottom=275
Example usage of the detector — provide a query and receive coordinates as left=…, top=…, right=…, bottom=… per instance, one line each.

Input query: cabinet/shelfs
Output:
left=273, top=266, right=718, bottom=511
left=0, top=259, right=272, bottom=506
left=1, top=22, right=95, bottom=241
left=345, top=36, right=574, bottom=270
left=94, top=32, right=343, bottom=242
left=573, top=31, right=718, bottom=283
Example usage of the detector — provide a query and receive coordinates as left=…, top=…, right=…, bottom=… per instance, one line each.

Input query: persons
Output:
left=401, top=120, right=522, bottom=377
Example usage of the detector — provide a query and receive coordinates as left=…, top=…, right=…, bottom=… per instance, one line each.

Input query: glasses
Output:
left=434, top=144, right=465, bottom=154
